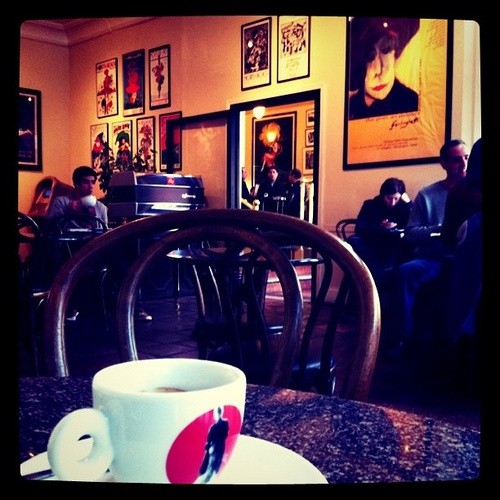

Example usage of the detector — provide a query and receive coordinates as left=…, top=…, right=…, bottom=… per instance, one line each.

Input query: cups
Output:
left=80, top=195, right=97, bottom=208
left=47, top=355, right=247, bottom=484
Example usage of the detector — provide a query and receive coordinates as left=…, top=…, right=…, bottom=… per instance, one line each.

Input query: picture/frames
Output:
left=112, top=120, right=133, bottom=172
left=159, top=111, right=182, bottom=172
left=122, top=49, right=145, bottom=117
left=95, top=58, right=118, bottom=118
left=90, top=123, right=108, bottom=175
left=149, top=44, right=171, bottom=110
left=18, top=88, right=42, bottom=172
left=277, top=16, right=311, bottom=83
left=241, top=17, right=272, bottom=91
left=306, top=109, right=314, bottom=127
left=303, top=147, right=313, bottom=175
left=306, top=129, right=314, bottom=146
left=343, top=16, right=453, bottom=170
left=253, top=111, right=297, bottom=187
left=137, top=116, right=156, bottom=172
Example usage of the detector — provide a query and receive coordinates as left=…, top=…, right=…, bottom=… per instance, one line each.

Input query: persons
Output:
left=47, top=166, right=154, bottom=322
left=257, top=166, right=285, bottom=213
left=242, top=166, right=259, bottom=209
left=283, top=169, right=307, bottom=219
left=344, top=177, right=415, bottom=267
left=408, top=136, right=484, bottom=383
left=378, top=139, right=472, bottom=359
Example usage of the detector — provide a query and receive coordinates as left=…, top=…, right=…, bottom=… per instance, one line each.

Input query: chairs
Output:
left=45, top=208, right=380, bottom=402
left=19, top=211, right=120, bottom=357
left=221, top=241, right=359, bottom=396
left=336, top=219, right=358, bottom=243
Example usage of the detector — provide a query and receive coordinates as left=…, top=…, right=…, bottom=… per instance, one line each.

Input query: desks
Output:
left=252, top=196, right=286, bottom=214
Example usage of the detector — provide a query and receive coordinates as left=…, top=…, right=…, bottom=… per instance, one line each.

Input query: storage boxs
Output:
left=108, top=172, right=205, bottom=216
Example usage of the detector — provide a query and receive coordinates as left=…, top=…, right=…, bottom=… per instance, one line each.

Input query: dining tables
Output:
left=167, top=248, right=325, bottom=362
left=20, top=377, right=480, bottom=484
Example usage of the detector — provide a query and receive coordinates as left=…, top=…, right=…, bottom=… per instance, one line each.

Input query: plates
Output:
left=44, top=433, right=329, bottom=484
